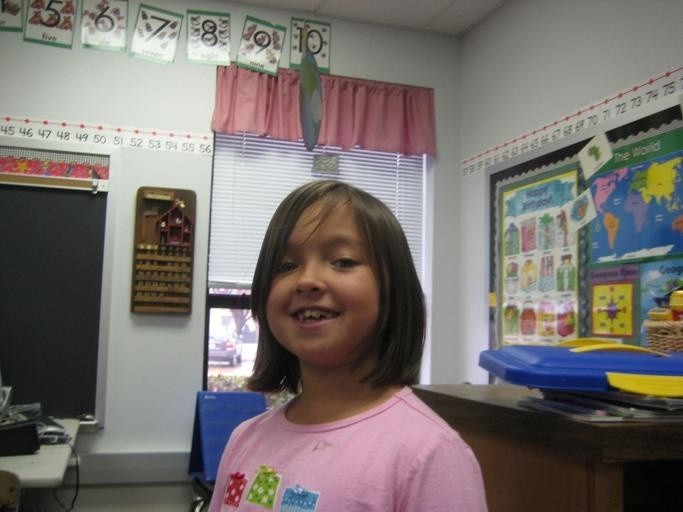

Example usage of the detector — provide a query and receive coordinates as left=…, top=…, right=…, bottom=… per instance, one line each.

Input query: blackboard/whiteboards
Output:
left=0, top=173, right=116, bottom=429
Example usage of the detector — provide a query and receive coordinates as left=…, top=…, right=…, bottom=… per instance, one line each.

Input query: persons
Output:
left=209, top=179, right=490, bottom=511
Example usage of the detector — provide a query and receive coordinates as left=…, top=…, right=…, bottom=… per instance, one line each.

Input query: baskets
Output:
left=643, top=319, right=683, bottom=352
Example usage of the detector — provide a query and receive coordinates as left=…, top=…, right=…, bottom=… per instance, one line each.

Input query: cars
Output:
left=208, top=336, right=242, bottom=367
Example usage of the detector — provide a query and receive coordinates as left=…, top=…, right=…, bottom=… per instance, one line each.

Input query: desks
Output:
left=411, top=384, right=683, bottom=512
left=0, top=417, right=80, bottom=488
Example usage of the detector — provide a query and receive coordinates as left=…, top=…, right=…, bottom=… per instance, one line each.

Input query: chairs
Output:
left=0, top=469, right=22, bottom=512
